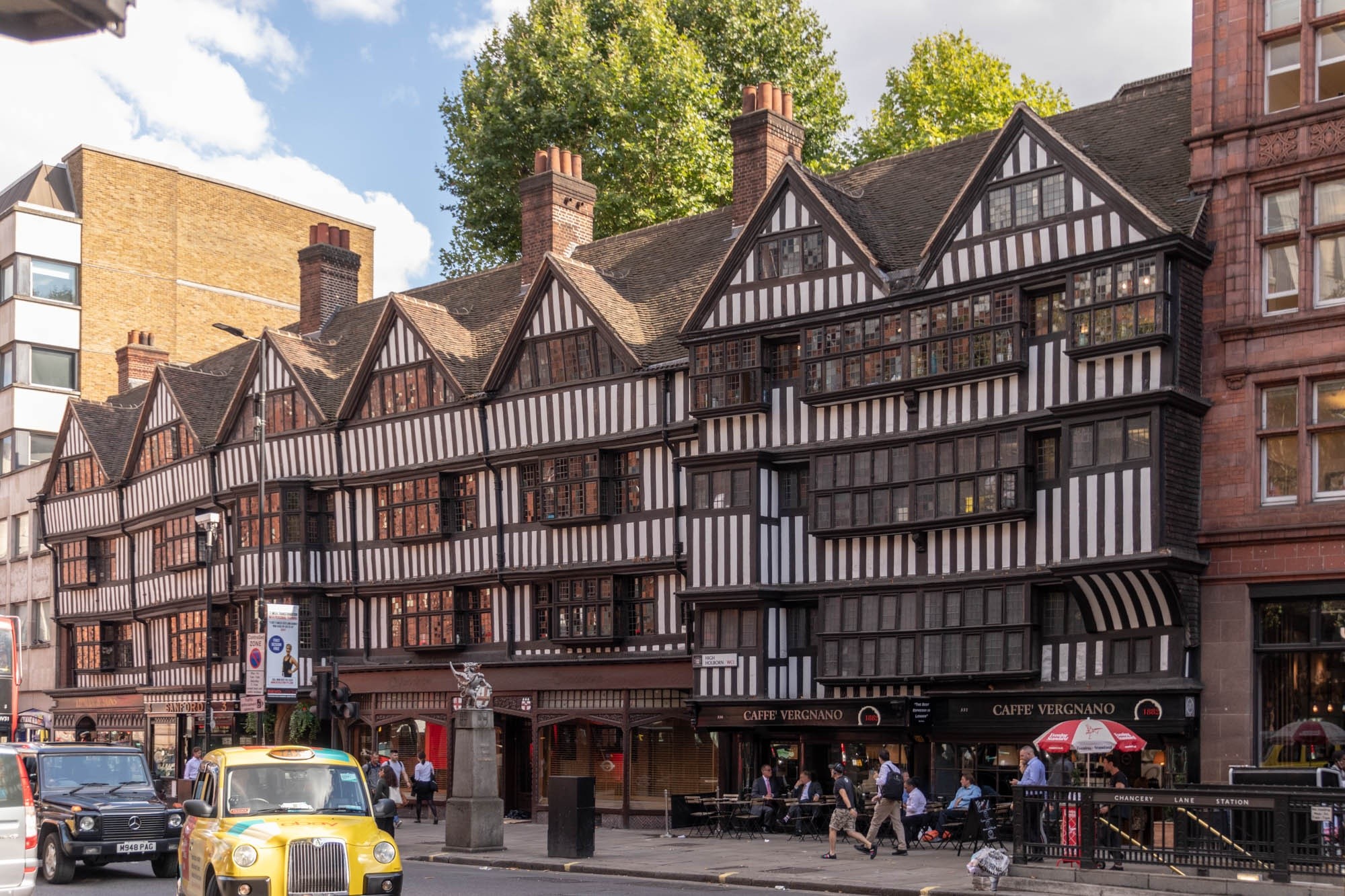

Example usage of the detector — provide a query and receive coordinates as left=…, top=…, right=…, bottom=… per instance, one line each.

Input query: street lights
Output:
left=193, top=501, right=225, bottom=754
left=212, top=323, right=266, bottom=634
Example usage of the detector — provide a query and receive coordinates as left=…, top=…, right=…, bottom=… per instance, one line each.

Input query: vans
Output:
left=0, top=742, right=40, bottom=896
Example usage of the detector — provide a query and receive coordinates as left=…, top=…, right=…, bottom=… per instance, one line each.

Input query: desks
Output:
left=703, top=802, right=754, bottom=841
left=797, top=802, right=836, bottom=843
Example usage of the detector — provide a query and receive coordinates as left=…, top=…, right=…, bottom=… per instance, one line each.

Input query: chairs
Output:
left=303, top=768, right=344, bottom=803
left=684, top=793, right=1014, bottom=852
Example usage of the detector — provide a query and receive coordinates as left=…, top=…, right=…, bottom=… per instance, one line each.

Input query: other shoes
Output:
left=796, top=829, right=802, bottom=836
left=414, top=820, right=421, bottom=823
left=434, top=817, right=438, bottom=824
left=776, top=819, right=786, bottom=826
left=854, top=844, right=870, bottom=854
left=1110, top=865, right=1124, bottom=871
left=395, top=820, right=402, bottom=828
left=892, top=849, right=908, bottom=856
left=1028, top=856, right=1044, bottom=863
left=761, top=826, right=769, bottom=834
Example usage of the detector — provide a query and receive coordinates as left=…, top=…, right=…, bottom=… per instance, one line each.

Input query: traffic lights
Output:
left=331, top=686, right=348, bottom=703
left=329, top=702, right=360, bottom=721
left=309, top=672, right=330, bottom=722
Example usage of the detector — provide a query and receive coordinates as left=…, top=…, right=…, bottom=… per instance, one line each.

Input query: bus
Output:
left=0, top=614, right=24, bottom=742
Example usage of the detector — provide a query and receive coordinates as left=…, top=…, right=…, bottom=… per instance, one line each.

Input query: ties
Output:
left=767, top=779, right=770, bottom=793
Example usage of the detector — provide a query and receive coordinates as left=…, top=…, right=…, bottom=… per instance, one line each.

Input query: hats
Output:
left=828, top=763, right=844, bottom=773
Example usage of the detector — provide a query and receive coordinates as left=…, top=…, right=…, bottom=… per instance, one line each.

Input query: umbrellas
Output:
left=1266, top=719, right=1345, bottom=763
left=1032, top=716, right=1146, bottom=786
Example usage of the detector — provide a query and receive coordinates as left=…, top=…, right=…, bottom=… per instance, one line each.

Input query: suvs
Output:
left=177, top=745, right=403, bottom=896
left=2, top=741, right=185, bottom=885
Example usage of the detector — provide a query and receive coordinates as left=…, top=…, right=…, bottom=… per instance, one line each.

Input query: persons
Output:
left=928, top=773, right=981, bottom=843
left=372, top=765, right=398, bottom=839
left=1009, top=745, right=1048, bottom=862
left=382, top=749, right=411, bottom=827
left=821, top=762, right=877, bottom=859
left=411, top=751, right=439, bottom=824
left=1097, top=754, right=1130, bottom=871
left=183, top=746, right=203, bottom=780
left=751, top=764, right=781, bottom=834
left=899, top=771, right=926, bottom=817
left=1330, top=751, right=1345, bottom=828
left=853, top=748, right=908, bottom=856
left=779, top=771, right=823, bottom=836
left=362, top=751, right=382, bottom=789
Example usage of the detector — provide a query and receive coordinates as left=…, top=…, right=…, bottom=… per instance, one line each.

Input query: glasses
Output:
left=763, top=769, right=773, bottom=771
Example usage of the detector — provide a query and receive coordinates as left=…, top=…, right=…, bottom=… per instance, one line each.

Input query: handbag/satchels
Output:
left=430, top=774, right=438, bottom=792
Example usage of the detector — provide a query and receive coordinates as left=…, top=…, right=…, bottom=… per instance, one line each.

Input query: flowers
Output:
left=289, top=702, right=321, bottom=747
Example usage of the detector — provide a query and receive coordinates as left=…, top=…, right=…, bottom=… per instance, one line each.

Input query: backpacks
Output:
left=879, top=764, right=904, bottom=800
left=850, top=782, right=866, bottom=813
left=382, top=783, right=402, bottom=804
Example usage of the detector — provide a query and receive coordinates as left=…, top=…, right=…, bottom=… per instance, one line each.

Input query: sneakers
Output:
left=821, top=851, right=836, bottom=859
left=870, top=844, right=877, bottom=859
